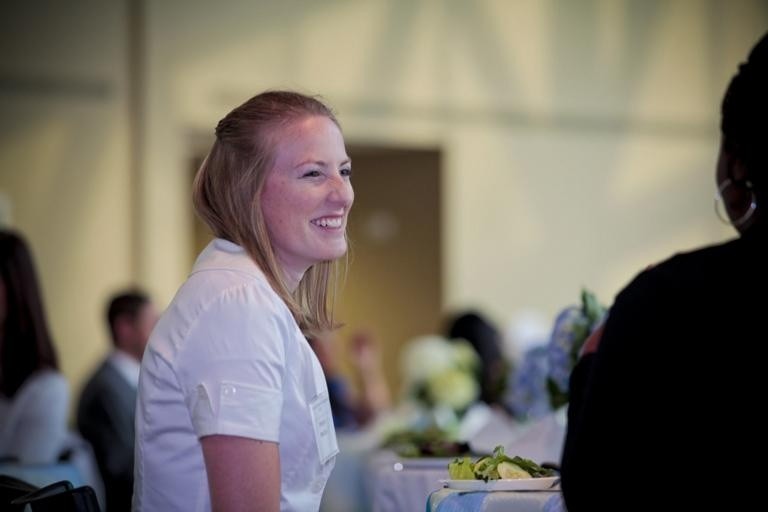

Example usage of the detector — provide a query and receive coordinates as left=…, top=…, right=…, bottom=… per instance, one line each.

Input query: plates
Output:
left=395, top=457, right=473, bottom=467
left=439, top=476, right=560, bottom=491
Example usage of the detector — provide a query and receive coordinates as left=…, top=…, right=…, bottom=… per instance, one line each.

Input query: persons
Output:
left=71, top=287, right=159, bottom=511
left=129, top=88, right=358, bottom=512
left=560, top=30, right=768, bottom=512
left=298, top=307, right=515, bottom=458
left=0, top=226, right=74, bottom=484
